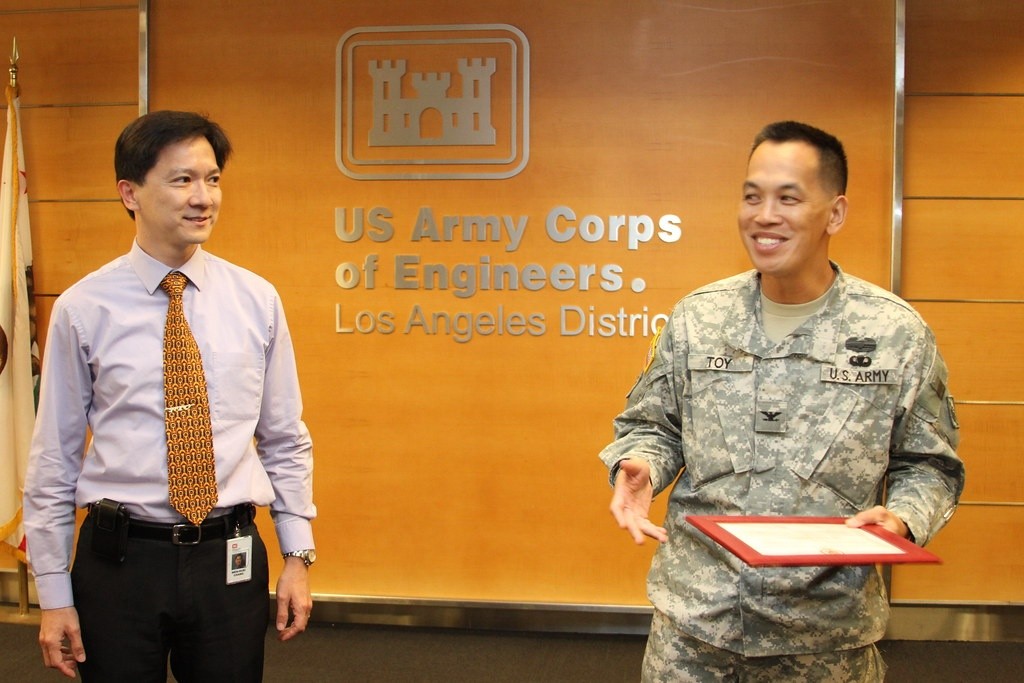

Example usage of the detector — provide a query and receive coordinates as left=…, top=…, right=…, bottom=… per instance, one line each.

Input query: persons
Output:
left=598, top=120, right=965, bottom=683
left=233, top=554, right=243, bottom=568
left=23, top=110, right=318, bottom=683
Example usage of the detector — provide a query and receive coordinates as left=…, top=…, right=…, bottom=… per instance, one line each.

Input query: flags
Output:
left=1, top=88, right=41, bottom=566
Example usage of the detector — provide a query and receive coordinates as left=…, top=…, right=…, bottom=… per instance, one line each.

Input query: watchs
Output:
left=283, top=549, right=317, bottom=566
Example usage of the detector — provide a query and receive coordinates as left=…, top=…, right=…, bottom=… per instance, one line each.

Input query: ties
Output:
left=159, top=272, right=218, bottom=526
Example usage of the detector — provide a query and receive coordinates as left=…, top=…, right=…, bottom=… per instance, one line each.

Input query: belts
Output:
left=88, top=503, right=257, bottom=546
